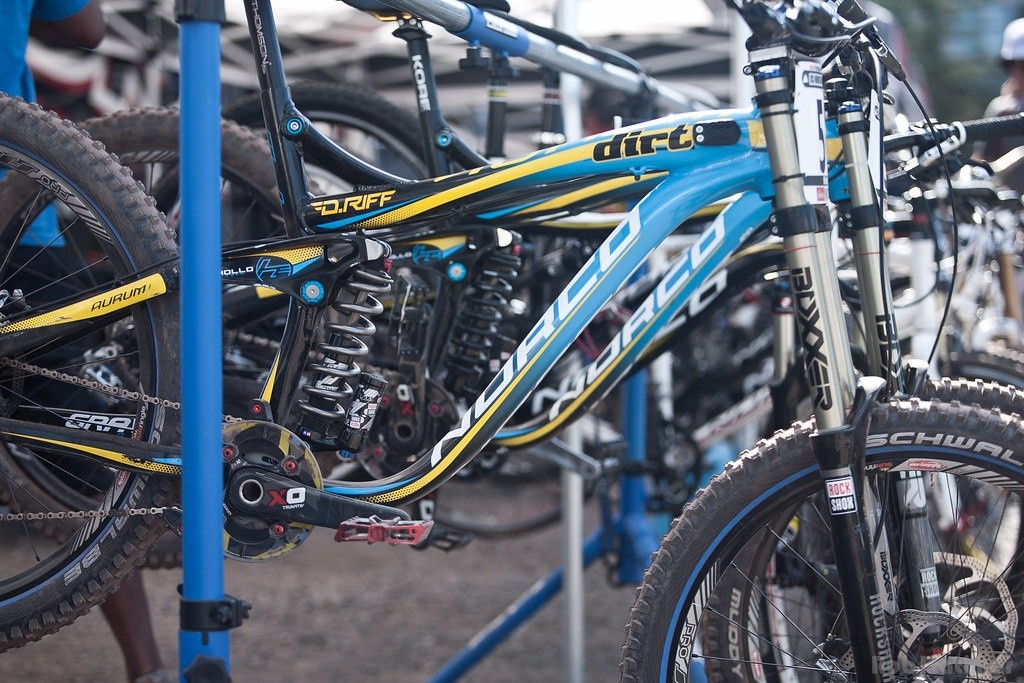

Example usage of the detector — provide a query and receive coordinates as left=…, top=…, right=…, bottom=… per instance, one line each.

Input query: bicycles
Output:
left=1, top=0, right=1024, bottom=683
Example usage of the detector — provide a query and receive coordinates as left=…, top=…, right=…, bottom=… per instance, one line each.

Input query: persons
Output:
left=968, top=15, right=1024, bottom=351
left=0, top=0, right=172, bottom=683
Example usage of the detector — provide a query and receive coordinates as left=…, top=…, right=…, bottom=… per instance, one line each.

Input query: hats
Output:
left=1000, top=18, right=1024, bottom=60
